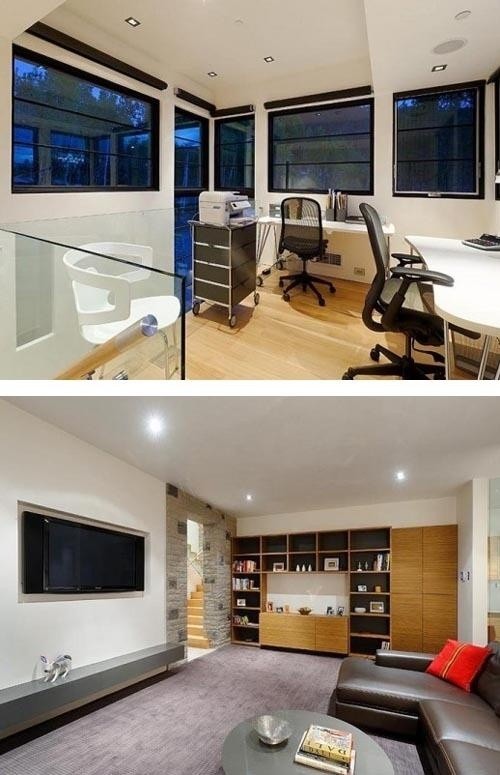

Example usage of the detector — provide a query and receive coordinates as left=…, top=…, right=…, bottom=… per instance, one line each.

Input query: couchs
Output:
left=327, top=641, right=500, bottom=774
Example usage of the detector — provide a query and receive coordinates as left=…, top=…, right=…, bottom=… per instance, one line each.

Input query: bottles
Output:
left=296, top=564, right=312, bottom=572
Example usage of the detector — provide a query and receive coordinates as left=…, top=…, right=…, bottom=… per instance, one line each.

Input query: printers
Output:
left=198, top=191, right=251, bottom=227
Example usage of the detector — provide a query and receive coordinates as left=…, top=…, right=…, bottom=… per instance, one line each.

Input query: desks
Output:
left=221, top=709, right=395, bottom=774
left=257, top=216, right=396, bottom=281
left=404, top=235, right=499, bottom=380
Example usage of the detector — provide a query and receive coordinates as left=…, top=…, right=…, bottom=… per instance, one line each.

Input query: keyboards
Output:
left=462, top=233, right=500, bottom=250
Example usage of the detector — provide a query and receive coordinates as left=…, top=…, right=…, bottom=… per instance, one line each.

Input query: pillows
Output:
left=426, top=638, right=492, bottom=694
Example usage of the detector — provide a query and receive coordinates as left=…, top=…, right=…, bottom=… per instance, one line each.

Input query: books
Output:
left=295, top=731, right=356, bottom=775
left=300, top=724, right=353, bottom=764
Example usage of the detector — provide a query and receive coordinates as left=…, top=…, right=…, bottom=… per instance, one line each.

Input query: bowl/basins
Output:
left=354, top=608, right=366, bottom=613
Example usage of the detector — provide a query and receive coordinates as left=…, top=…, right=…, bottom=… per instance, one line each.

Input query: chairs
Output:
left=342, top=203, right=480, bottom=380
left=63, top=240, right=182, bottom=381
left=279, top=197, right=337, bottom=306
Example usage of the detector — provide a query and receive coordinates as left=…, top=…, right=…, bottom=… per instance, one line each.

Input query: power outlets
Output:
left=354, top=266, right=366, bottom=277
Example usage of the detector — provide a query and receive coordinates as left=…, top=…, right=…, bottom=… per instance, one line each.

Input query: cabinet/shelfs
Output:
left=390, top=525, right=459, bottom=653
left=187, top=218, right=258, bottom=327
left=231, top=527, right=390, bottom=658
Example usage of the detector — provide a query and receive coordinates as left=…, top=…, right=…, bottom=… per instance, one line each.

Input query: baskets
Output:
left=298, top=610, right=311, bottom=615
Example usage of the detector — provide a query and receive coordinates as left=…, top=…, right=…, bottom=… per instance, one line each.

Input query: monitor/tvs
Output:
left=22, top=511, right=144, bottom=593
